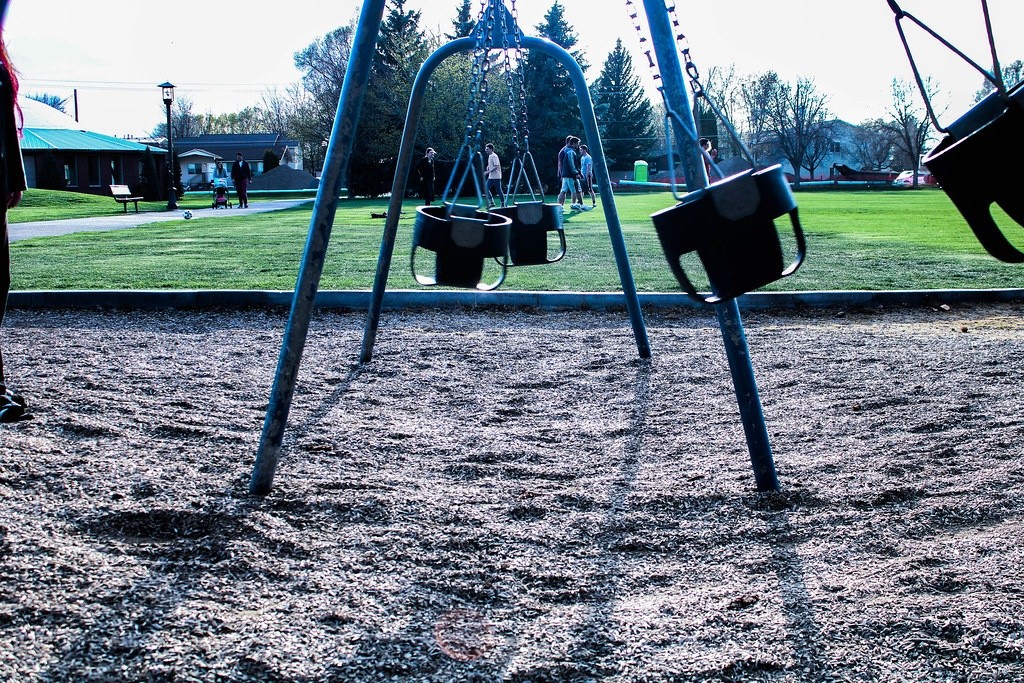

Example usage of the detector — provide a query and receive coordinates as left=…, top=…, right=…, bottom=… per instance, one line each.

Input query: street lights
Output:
left=156, top=80, right=183, bottom=211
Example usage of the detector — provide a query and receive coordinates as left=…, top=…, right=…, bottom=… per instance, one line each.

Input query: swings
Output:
left=886, top=0, right=1024, bottom=264
left=488, top=0, right=567, bottom=267
left=410, top=1, right=513, bottom=291
left=622, top=0, right=807, bottom=305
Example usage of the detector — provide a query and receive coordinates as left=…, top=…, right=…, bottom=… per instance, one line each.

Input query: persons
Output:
left=485, top=143, right=505, bottom=208
left=575, top=145, right=597, bottom=208
left=213, top=161, right=228, bottom=178
left=230, top=153, right=253, bottom=208
left=700, top=138, right=716, bottom=177
left=558, top=136, right=577, bottom=208
left=420, top=148, right=437, bottom=206
left=557, top=138, right=587, bottom=209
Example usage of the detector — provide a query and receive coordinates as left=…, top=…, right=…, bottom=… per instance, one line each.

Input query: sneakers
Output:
left=570, top=203, right=596, bottom=212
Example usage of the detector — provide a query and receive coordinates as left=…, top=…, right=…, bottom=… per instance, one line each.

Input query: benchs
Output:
left=109, top=184, right=144, bottom=214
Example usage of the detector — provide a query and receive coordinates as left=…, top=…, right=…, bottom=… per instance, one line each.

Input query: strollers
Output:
left=211, top=177, right=234, bottom=210
